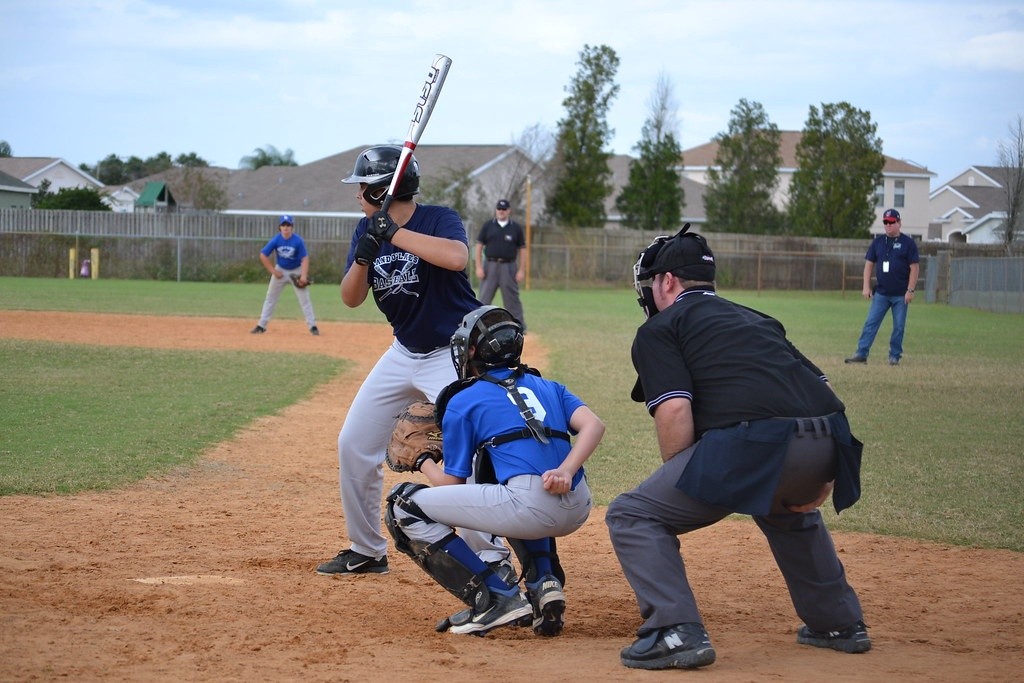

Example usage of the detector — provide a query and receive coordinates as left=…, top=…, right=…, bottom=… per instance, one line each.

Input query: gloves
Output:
left=352, top=219, right=383, bottom=265
left=368, top=210, right=399, bottom=244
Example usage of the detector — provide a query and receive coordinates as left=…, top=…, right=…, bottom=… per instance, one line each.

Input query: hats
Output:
left=497, top=199, right=510, bottom=209
left=882, top=208, right=900, bottom=221
left=279, top=216, right=294, bottom=226
left=636, top=237, right=714, bottom=281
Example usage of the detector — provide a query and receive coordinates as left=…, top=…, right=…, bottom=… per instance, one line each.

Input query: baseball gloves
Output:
left=289, top=272, right=314, bottom=289
left=385, top=399, right=444, bottom=475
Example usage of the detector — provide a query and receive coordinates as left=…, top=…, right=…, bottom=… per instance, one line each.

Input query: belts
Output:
left=739, top=418, right=833, bottom=432
left=486, top=257, right=514, bottom=263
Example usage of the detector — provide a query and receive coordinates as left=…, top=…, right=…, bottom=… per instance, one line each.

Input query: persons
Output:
left=385, top=305, right=606, bottom=637
left=475, top=199, right=527, bottom=336
left=844, top=209, right=919, bottom=365
left=250, top=215, right=320, bottom=336
left=607, top=231, right=872, bottom=668
left=316, top=142, right=486, bottom=575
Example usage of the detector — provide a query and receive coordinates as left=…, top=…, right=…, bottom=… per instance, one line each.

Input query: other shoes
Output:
left=250, top=326, right=267, bottom=335
left=311, top=326, right=320, bottom=335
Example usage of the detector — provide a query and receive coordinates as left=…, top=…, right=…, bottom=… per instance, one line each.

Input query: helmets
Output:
left=343, top=146, right=421, bottom=204
left=474, top=309, right=524, bottom=367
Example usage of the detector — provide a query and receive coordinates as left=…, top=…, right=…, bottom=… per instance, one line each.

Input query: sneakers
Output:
left=316, top=549, right=390, bottom=577
left=435, top=584, right=534, bottom=635
left=889, top=357, right=898, bottom=365
left=843, top=357, right=867, bottom=365
left=528, top=578, right=566, bottom=637
left=795, top=624, right=871, bottom=653
left=620, top=622, right=715, bottom=670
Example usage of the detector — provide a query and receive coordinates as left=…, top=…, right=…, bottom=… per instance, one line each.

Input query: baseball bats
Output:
left=357, top=51, right=454, bottom=268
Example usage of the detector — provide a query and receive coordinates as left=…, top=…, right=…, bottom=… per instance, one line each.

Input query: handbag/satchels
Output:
left=676, top=422, right=794, bottom=520
left=830, top=414, right=864, bottom=516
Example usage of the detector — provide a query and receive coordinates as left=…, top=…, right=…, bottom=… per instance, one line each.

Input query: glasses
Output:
left=883, top=220, right=896, bottom=225
left=496, top=207, right=507, bottom=211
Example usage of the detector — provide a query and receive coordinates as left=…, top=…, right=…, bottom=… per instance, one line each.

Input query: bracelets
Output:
left=907, top=288, right=914, bottom=292
